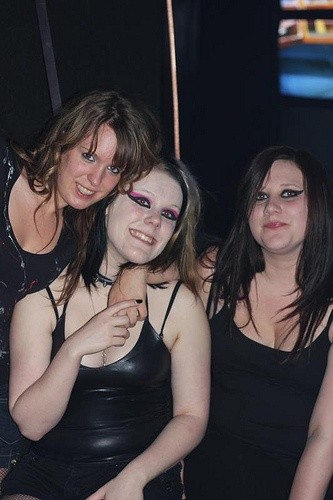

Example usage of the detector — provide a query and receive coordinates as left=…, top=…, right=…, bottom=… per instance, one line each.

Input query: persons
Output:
left=0, top=89, right=197, bottom=488
left=192, top=144, right=332, bottom=500
left=1, top=153, right=212, bottom=500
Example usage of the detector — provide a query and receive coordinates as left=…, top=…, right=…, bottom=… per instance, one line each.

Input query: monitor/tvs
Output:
left=273, top=10, right=333, bottom=105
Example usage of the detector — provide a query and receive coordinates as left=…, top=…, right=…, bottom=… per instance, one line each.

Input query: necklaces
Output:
left=94, top=270, right=116, bottom=287
left=86, top=281, right=108, bottom=366
